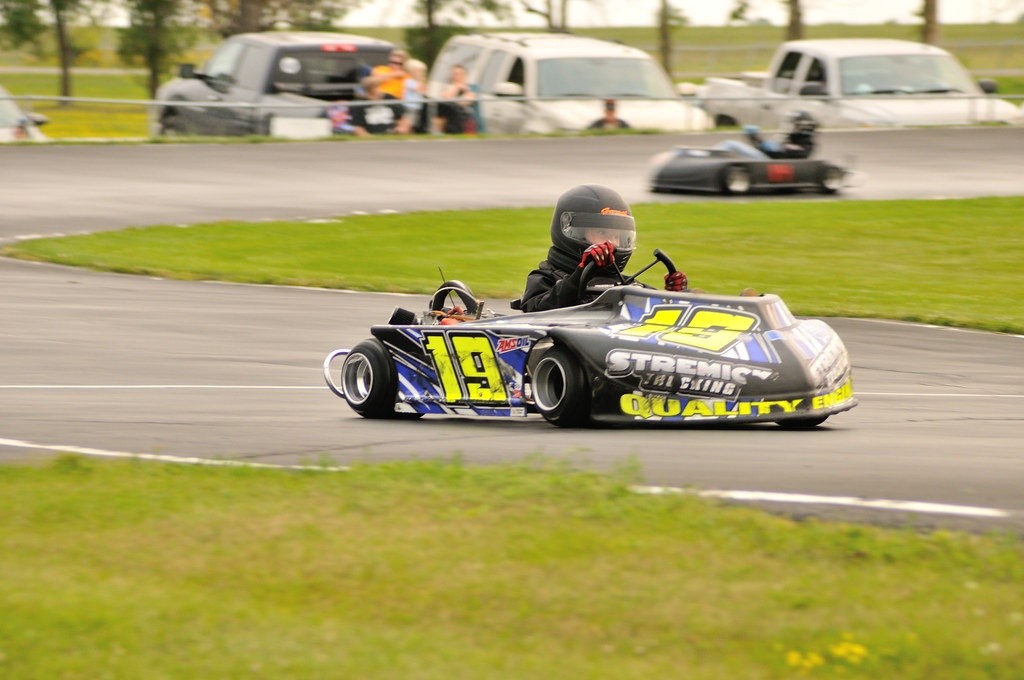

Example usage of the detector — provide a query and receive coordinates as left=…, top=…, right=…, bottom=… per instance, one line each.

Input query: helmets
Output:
left=550, top=183, right=637, bottom=274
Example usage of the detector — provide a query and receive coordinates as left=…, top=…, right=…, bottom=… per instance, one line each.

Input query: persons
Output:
left=521, top=185, right=687, bottom=312
left=744, top=111, right=819, bottom=160
left=347, top=49, right=474, bottom=135
left=584, top=99, right=629, bottom=129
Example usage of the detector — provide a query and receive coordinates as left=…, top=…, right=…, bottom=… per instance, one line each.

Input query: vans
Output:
left=423, top=29, right=714, bottom=134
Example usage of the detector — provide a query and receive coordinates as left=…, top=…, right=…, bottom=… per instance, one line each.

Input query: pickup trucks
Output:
left=705, top=37, right=1022, bottom=128
left=150, top=28, right=443, bottom=143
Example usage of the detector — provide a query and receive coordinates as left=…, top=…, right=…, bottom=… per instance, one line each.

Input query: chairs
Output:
left=465, top=85, right=487, bottom=133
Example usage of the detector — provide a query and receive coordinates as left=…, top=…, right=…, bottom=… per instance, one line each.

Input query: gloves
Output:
left=578, top=240, right=616, bottom=270
left=664, top=270, right=688, bottom=293
